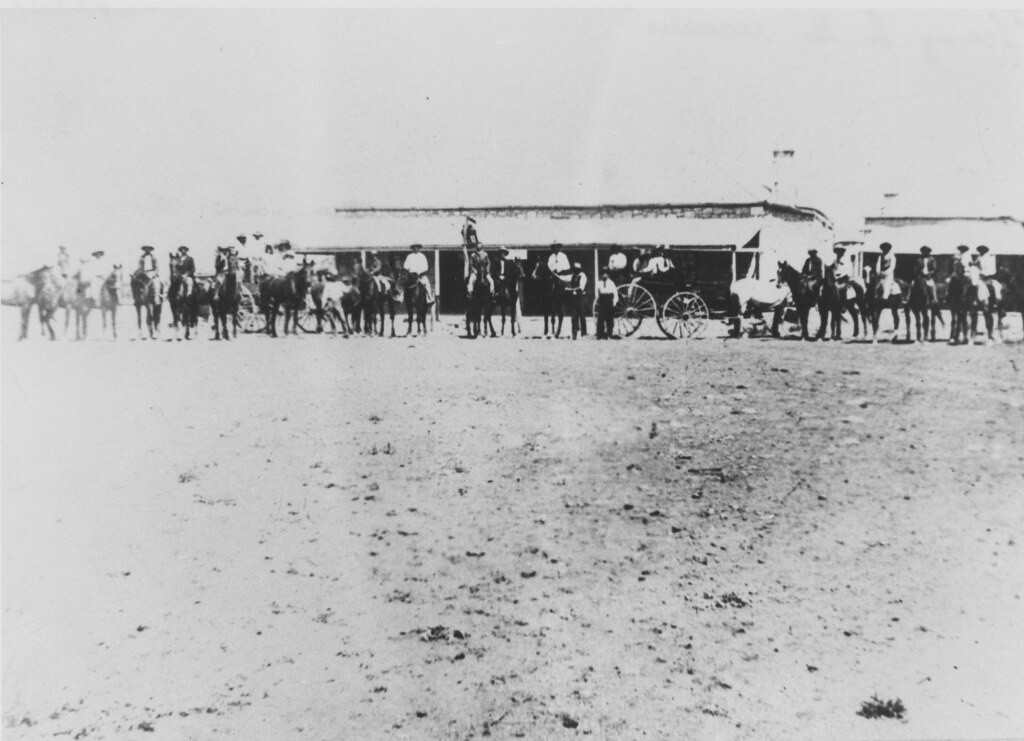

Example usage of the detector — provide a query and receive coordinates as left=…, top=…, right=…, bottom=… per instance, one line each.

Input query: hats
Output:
left=410, top=244, right=422, bottom=249
left=253, top=231, right=263, bottom=237
left=920, top=246, right=931, bottom=251
left=834, top=244, right=846, bottom=252
left=549, top=240, right=564, bottom=249
left=808, top=247, right=817, bottom=254
left=497, top=246, right=509, bottom=255
left=92, top=251, right=104, bottom=255
left=178, top=247, right=189, bottom=251
left=142, top=245, right=154, bottom=250
left=275, top=240, right=291, bottom=250
left=977, top=245, right=989, bottom=252
left=958, top=245, right=969, bottom=251
left=880, top=242, right=892, bottom=250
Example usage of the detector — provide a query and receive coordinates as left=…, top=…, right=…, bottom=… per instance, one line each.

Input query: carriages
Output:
left=236, top=255, right=373, bottom=336
left=594, top=241, right=792, bottom=338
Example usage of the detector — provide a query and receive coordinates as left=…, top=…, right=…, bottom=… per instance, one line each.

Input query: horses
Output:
left=167, top=251, right=243, bottom=342
left=775, top=260, right=867, bottom=340
left=13, top=265, right=96, bottom=341
left=465, top=257, right=526, bottom=339
left=99, top=264, right=166, bottom=341
left=351, top=265, right=434, bottom=339
left=860, top=260, right=1007, bottom=345
left=531, top=257, right=588, bottom=340
left=260, top=259, right=360, bottom=338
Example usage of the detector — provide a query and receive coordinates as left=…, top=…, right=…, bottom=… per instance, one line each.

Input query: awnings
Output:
left=295, top=210, right=761, bottom=325
left=863, top=219, right=1024, bottom=255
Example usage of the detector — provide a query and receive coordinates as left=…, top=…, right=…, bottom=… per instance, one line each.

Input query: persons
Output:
left=57, top=242, right=675, bottom=339
left=800, top=242, right=998, bottom=325
